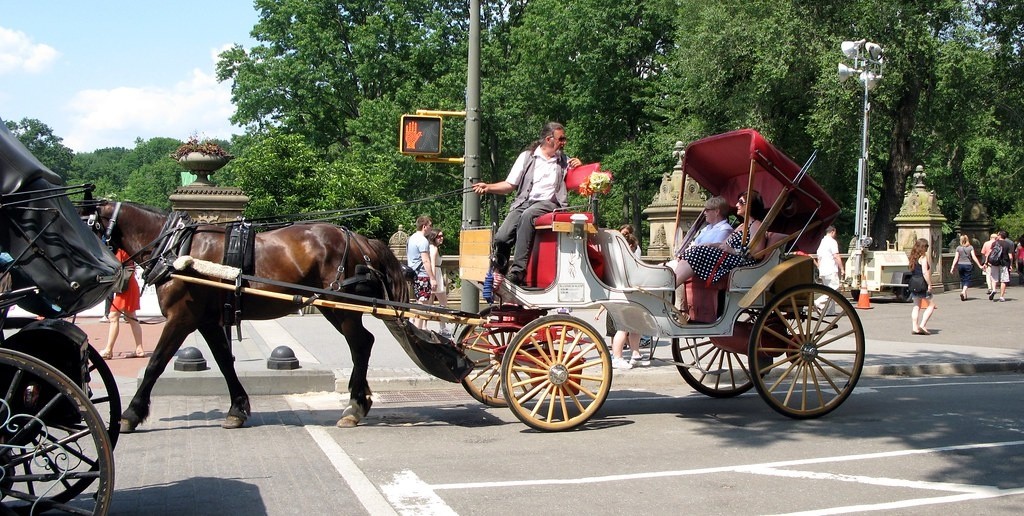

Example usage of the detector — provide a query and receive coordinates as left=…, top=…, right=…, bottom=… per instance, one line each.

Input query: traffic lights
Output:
left=398, top=114, right=441, bottom=156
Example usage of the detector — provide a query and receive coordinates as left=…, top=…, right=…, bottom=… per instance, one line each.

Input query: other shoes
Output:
left=826, top=311, right=838, bottom=316
left=912, top=329, right=923, bottom=335
left=919, top=325, right=930, bottom=336
left=629, top=356, right=651, bottom=366
left=814, top=300, right=822, bottom=316
left=960, top=292, right=968, bottom=300
left=100, top=316, right=110, bottom=323
left=989, top=289, right=997, bottom=300
left=611, top=357, right=632, bottom=369
left=1000, top=296, right=1006, bottom=302
left=506, top=271, right=519, bottom=286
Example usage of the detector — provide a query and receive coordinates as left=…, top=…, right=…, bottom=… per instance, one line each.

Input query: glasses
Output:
left=737, top=199, right=747, bottom=205
left=705, top=207, right=716, bottom=211
left=436, top=235, right=443, bottom=238
left=550, top=137, right=567, bottom=143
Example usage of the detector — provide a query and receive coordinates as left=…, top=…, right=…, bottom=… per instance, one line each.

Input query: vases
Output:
left=178, top=151, right=228, bottom=188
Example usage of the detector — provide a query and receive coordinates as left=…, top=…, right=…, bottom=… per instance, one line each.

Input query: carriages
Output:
left=75, top=126, right=865, bottom=435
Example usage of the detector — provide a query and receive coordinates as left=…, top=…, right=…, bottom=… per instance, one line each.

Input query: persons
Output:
left=405, top=216, right=452, bottom=338
left=981, top=231, right=1024, bottom=302
left=950, top=234, right=982, bottom=301
left=594, top=223, right=651, bottom=369
left=100, top=292, right=130, bottom=323
left=473, top=122, right=582, bottom=286
left=673, top=189, right=766, bottom=284
left=98, top=248, right=145, bottom=359
left=906, top=238, right=936, bottom=334
left=659, top=194, right=734, bottom=320
left=812, top=222, right=845, bottom=316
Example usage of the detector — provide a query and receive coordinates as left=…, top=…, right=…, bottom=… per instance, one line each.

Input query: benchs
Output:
left=686, top=231, right=788, bottom=291
left=596, top=227, right=676, bottom=306
left=532, top=163, right=601, bottom=231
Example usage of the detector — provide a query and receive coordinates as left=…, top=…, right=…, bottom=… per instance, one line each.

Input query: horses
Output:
left=69, top=199, right=410, bottom=434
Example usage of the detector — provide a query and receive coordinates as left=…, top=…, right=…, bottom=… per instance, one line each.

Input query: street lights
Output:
left=837, top=39, right=885, bottom=279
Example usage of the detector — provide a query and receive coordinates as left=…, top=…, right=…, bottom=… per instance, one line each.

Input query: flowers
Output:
left=169, top=139, right=235, bottom=164
left=579, top=169, right=615, bottom=199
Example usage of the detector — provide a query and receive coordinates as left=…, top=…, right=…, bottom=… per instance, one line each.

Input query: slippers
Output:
left=123, top=350, right=146, bottom=358
left=99, top=351, right=111, bottom=359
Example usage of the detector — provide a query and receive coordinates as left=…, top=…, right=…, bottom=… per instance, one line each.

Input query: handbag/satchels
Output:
left=908, top=275, right=928, bottom=292
left=400, top=263, right=416, bottom=281
left=988, top=243, right=1001, bottom=264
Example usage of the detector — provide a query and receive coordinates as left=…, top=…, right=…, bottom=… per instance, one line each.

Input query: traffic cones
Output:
left=853, top=277, right=874, bottom=310
